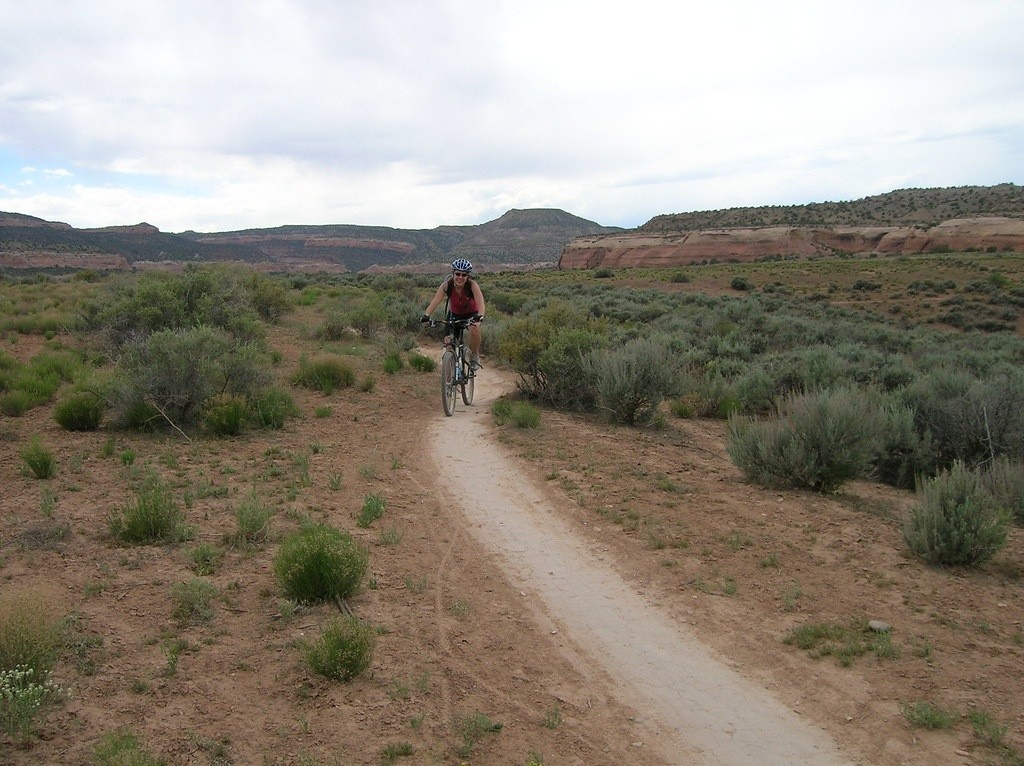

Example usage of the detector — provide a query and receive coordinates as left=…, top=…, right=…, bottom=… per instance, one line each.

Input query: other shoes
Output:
left=470, top=353, right=480, bottom=372
left=446, top=383, right=452, bottom=396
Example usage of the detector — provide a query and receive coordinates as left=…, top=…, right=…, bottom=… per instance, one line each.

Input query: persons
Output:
left=419, top=258, right=486, bottom=385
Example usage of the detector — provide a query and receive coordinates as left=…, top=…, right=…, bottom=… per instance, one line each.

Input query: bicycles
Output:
left=424, top=314, right=483, bottom=416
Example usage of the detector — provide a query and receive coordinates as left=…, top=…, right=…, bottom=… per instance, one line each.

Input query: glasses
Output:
left=454, top=273, right=467, bottom=277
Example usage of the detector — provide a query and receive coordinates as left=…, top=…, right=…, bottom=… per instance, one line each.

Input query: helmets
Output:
left=452, top=258, right=473, bottom=273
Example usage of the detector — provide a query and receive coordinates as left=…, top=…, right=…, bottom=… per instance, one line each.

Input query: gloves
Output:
left=419, top=314, right=430, bottom=323
left=473, top=314, right=482, bottom=322
